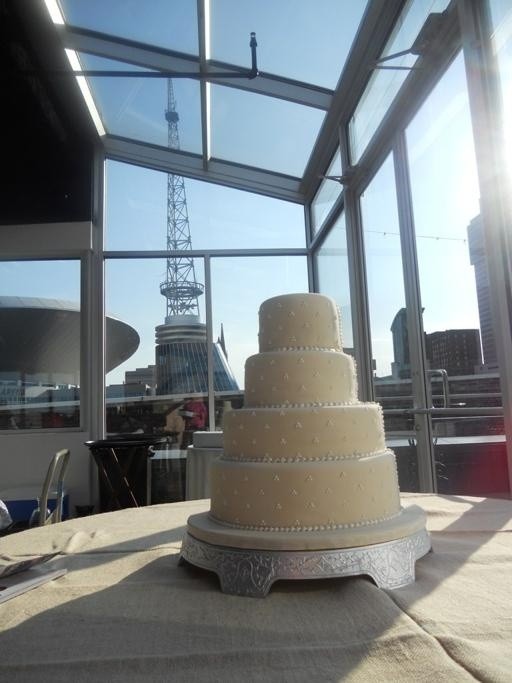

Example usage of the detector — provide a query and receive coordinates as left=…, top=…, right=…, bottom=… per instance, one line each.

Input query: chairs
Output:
left=1, top=447, right=72, bottom=540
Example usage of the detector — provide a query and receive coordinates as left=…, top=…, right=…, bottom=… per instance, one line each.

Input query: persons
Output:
left=1, top=391, right=208, bottom=477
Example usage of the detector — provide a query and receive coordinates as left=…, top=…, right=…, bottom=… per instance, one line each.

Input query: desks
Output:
left=84, top=438, right=167, bottom=510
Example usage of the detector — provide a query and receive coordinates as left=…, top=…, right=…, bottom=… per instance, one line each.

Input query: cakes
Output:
left=207, top=292, right=402, bottom=534
